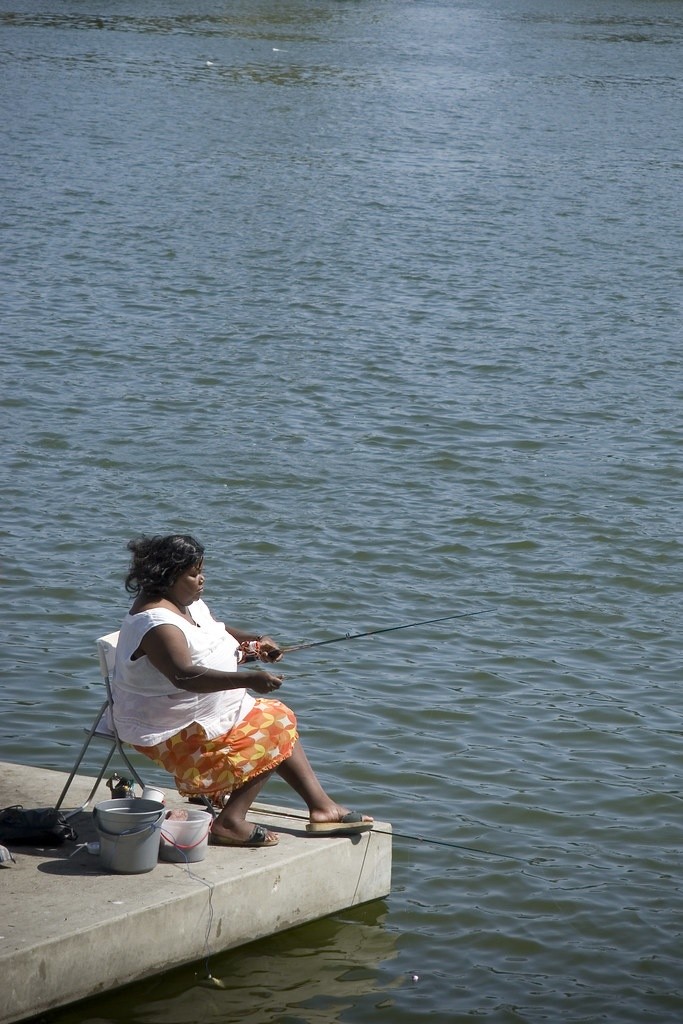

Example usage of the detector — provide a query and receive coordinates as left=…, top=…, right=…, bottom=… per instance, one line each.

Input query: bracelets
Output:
left=256, top=635, right=264, bottom=641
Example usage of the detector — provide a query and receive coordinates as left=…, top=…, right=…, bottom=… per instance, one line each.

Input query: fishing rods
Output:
left=261, top=608, right=500, bottom=657
left=188, top=793, right=542, bottom=866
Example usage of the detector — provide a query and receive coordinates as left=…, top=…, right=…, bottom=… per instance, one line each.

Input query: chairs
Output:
left=55, top=631, right=217, bottom=820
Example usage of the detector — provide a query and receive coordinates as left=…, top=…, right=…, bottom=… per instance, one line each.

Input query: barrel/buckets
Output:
left=159, top=807, right=212, bottom=863
left=93, top=798, right=166, bottom=873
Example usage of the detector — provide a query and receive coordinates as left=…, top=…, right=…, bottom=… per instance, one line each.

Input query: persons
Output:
left=111, top=534, right=373, bottom=846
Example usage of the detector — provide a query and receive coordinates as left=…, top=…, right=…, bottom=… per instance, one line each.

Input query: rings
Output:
left=271, top=689, right=273, bottom=692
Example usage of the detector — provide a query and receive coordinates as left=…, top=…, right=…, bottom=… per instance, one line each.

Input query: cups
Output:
left=141, top=786, right=166, bottom=805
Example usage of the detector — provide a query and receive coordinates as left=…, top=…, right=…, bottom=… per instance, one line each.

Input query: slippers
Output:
left=210, top=824, right=279, bottom=846
left=305, top=810, right=373, bottom=834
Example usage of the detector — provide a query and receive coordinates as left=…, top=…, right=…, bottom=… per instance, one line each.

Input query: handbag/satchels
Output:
left=106, top=775, right=134, bottom=799
left=0, top=804, right=78, bottom=849
